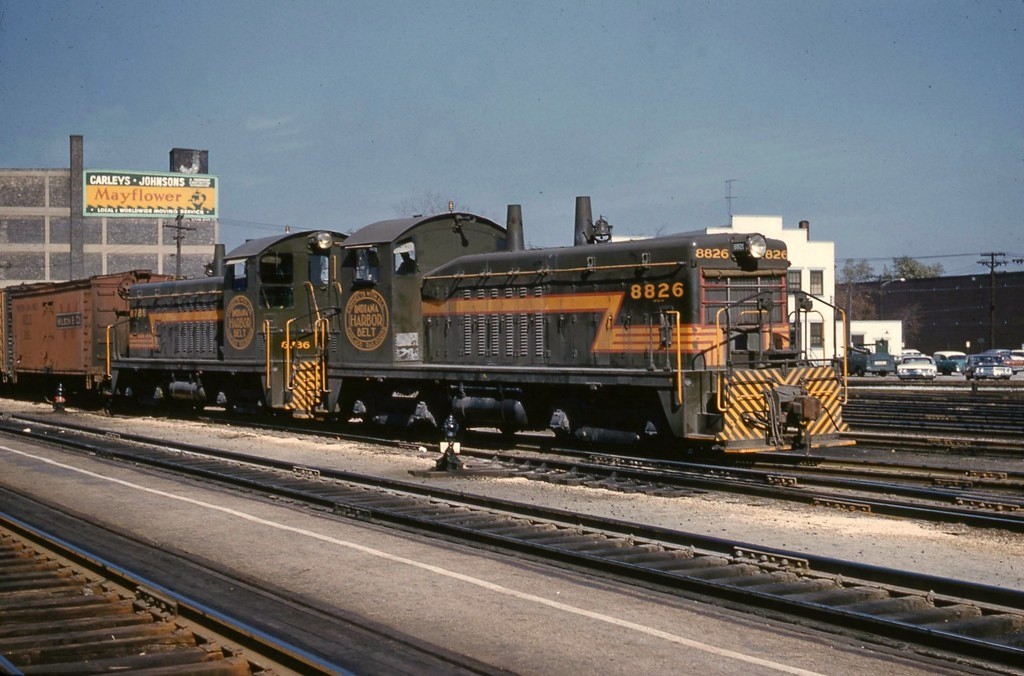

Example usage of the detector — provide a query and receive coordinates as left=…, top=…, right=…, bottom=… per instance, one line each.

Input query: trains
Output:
left=0, top=196, right=858, bottom=459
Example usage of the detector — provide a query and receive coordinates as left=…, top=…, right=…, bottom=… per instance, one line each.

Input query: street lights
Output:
left=963, top=337, right=972, bottom=354
left=878, top=274, right=906, bottom=320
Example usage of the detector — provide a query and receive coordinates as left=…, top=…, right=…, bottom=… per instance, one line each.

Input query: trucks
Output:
left=832, top=344, right=895, bottom=377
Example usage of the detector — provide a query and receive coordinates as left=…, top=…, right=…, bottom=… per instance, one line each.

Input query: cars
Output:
left=894, top=349, right=921, bottom=361
left=961, top=354, right=1012, bottom=380
left=933, top=350, right=966, bottom=374
left=976, top=348, right=1010, bottom=357
left=896, top=356, right=937, bottom=380
left=1011, top=350, right=1024, bottom=361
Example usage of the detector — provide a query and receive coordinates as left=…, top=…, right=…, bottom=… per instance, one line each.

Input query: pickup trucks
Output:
left=998, top=354, right=1024, bottom=375
